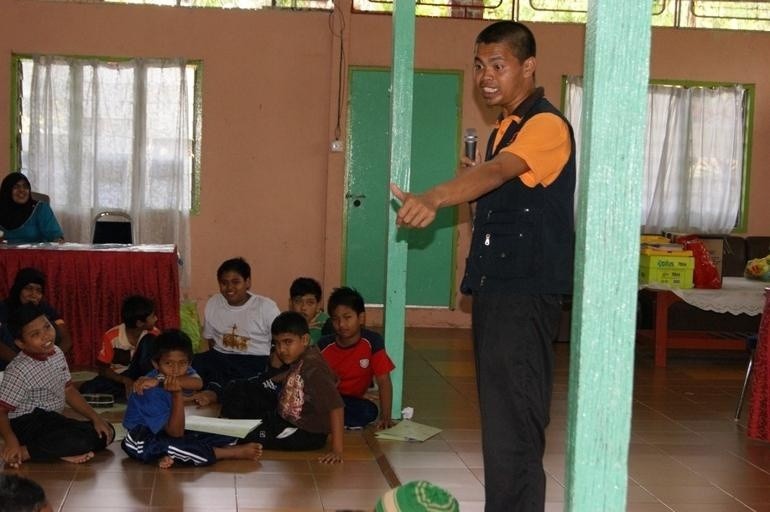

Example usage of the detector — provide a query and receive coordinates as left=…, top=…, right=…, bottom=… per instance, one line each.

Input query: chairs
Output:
left=693, top=233, right=770, bottom=278
left=88, top=211, right=134, bottom=245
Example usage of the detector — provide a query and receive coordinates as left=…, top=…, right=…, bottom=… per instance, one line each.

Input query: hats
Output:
left=373, top=481, right=460, bottom=512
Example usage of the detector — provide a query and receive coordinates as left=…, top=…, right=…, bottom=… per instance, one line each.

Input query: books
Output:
left=184, top=415, right=263, bottom=439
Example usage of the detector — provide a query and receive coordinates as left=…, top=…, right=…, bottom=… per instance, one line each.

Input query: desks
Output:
left=639, top=276, right=768, bottom=368
left=2, top=243, right=179, bottom=366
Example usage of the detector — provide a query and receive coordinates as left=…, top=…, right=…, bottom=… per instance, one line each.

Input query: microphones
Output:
left=463, top=128, right=478, bottom=168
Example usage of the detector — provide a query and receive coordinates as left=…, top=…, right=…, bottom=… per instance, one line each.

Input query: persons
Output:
left=78, top=294, right=162, bottom=407
left=192, top=257, right=280, bottom=390
left=0, top=267, right=73, bottom=387
left=390, top=22, right=575, bottom=512
left=0, top=474, right=53, bottom=512
left=0, top=303, right=116, bottom=469
left=288, top=277, right=334, bottom=344
left=190, top=287, right=398, bottom=430
left=217, top=310, right=346, bottom=464
left=0, top=171, right=64, bottom=245
left=121, top=329, right=263, bottom=470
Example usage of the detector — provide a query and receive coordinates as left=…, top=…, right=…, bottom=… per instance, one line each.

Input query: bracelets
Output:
left=156, top=373, right=165, bottom=387
